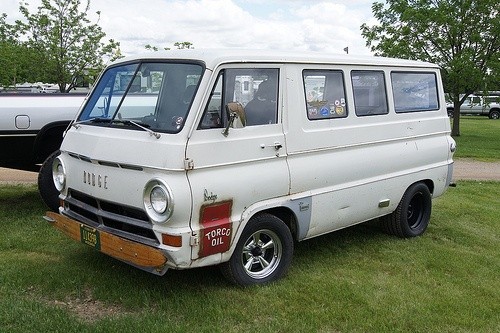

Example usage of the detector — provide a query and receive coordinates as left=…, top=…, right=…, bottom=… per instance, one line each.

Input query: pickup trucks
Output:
left=446, top=94, right=500, bottom=119
left=3, top=72, right=254, bottom=211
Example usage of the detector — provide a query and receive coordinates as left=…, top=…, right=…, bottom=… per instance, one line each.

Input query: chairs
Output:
left=244, top=80, right=278, bottom=125
left=177, top=84, right=197, bottom=118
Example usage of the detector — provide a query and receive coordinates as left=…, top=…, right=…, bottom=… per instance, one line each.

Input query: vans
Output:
left=43, top=44, right=457, bottom=286
